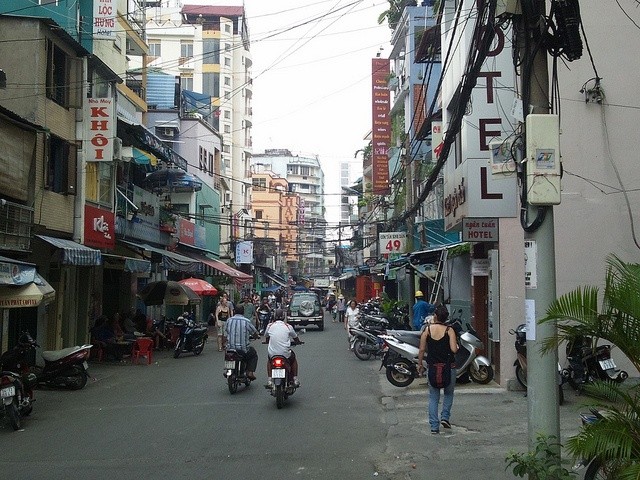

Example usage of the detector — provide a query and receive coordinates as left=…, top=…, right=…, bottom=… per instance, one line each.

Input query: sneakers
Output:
left=248, top=371, right=256, bottom=380
left=441, top=420, right=451, bottom=428
left=267, top=380, right=273, bottom=386
left=431, top=429, right=439, bottom=434
left=293, top=378, right=301, bottom=386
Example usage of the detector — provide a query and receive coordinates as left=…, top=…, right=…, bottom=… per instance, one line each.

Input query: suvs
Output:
left=286, top=293, right=324, bottom=331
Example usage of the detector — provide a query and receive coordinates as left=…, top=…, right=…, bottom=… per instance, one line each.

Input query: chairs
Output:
left=91, top=329, right=107, bottom=362
left=132, top=339, right=153, bottom=365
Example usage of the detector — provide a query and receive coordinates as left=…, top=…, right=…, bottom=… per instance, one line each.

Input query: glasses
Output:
left=244, top=298, right=249, bottom=299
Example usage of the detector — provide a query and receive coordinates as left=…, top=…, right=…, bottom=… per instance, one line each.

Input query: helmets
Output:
left=414, top=291, right=424, bottom=296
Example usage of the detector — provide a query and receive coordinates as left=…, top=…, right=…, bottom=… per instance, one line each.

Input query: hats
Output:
left=262, top=297, right=269, bottom=300
left=338, top=294, right=345, bottom=299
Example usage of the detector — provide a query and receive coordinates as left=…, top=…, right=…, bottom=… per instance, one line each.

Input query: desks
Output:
left=108, top=340, right=130, bottom=364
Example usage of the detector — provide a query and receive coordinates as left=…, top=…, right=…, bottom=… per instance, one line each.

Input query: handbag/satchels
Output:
left=429, top=350, right=453, bottom=388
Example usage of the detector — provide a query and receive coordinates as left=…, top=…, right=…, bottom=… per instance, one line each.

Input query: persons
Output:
left=344, top=300, right=361, bottom=350
left=332, top=290, right=346, bottom=323
left=418, top=305, right=459, bottom=434
left=240, top=287, right=290, bottom=323
left=91, top=309, right=172, bottom=364
left=264, top=310, right=302, bottom=386
left=216, top=292, right=233, bottom=351
left=413, top=291, right=436, bottom=331
left=223, top=304, right=259, bottom=380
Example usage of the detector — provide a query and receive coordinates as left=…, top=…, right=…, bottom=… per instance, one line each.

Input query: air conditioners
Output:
left=164, top=128, right=175, bottom=137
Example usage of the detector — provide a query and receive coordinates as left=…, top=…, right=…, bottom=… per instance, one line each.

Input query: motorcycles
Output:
left=348, top=300, right=410, bottom=361
left=2, top=330, right=93, bottom=389
left=262, top=328, right=306, bottom=409
left=508, top=324, right=570, bottom=406
left=378, top=309, right=494, bottom=387
left=580, top=408, right=640, bottom=480
left=1, top=346, right=36, bottom=430
left=560, top=325, right=629, bottom=402
left=256, top=310, right=271, bottom=335
left=173, top=316, right=208, bottom=359
left=219, top=328, right=263, bottom=394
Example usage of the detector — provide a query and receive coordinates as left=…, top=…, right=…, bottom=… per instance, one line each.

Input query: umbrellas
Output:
left=144, top=167, right=202, bottom=194
left=118, top=145, right=157, bottom=220
left=142, top=281, right=202, bottom=306
left=1, top=272, right=56, bottom=309
left=178, top=277, right=217, bottom=296
